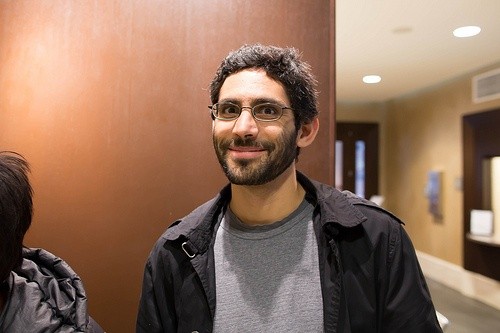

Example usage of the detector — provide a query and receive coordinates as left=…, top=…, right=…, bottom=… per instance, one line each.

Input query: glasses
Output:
left=207, top=102, right=295, bottom=122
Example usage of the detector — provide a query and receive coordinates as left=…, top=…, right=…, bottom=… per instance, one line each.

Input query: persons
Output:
left=136, top=44, right=445, bottom=332
left=1, top=149, right=104, bottom=332
